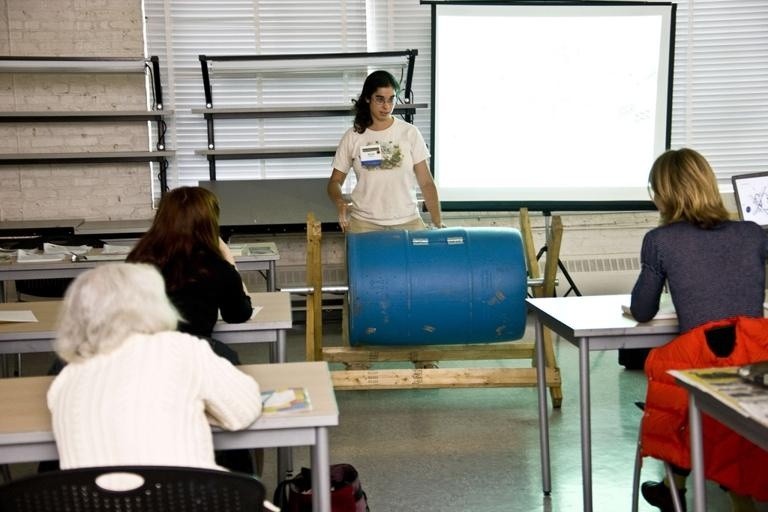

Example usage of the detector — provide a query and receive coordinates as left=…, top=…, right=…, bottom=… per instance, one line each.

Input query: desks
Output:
left=666, top=366, right=768, bottom=512
left=1, top=361, right=338, bottom=512
left=1, top=289, right=296, bottom=357
left=1, top=244, right=281, bottom=293
left=525, top=293, right=679, bottom=512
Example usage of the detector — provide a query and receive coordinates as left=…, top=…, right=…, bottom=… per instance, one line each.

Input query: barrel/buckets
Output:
left=346, top=226, right=526, bottom=348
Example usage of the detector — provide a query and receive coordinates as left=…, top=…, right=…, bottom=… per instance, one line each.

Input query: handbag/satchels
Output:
left=273, top=464, right=370, bottom=512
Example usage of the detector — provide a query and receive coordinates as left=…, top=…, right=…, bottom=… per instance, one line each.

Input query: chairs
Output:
left=632, top=317, right=768, bottom=512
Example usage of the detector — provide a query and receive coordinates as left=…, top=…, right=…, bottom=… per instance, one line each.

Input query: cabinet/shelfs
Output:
left=0, top=56, right=174, bottom=242
left=192, top=49, right=428, bottom=244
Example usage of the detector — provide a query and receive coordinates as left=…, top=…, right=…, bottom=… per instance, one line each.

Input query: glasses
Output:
left=371, top=97, right=397, bottom=104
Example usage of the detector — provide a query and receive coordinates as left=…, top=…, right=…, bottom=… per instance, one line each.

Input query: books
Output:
left=620, top=293, right=678, bottom=320
left=0, top=237, right=137, bottom=262
left=259, top=386, right=312, bottom=417
left=737, top=355, right=767, bottom=387
left=217, top=304, right=263, bottom=321
left=0, top=309, right=40, bottom=323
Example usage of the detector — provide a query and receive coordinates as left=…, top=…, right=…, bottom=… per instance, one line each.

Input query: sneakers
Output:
left=642, top=480, right=687, bottom=512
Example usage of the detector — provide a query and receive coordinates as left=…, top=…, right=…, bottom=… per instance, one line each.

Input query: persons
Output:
left=46, top=261, right=262, bottom=479
left=327, top=69, right=449, bottom=372
left=630, top=147, right=767, bottom=510
left=122, top=187, right=253, bottom=366
